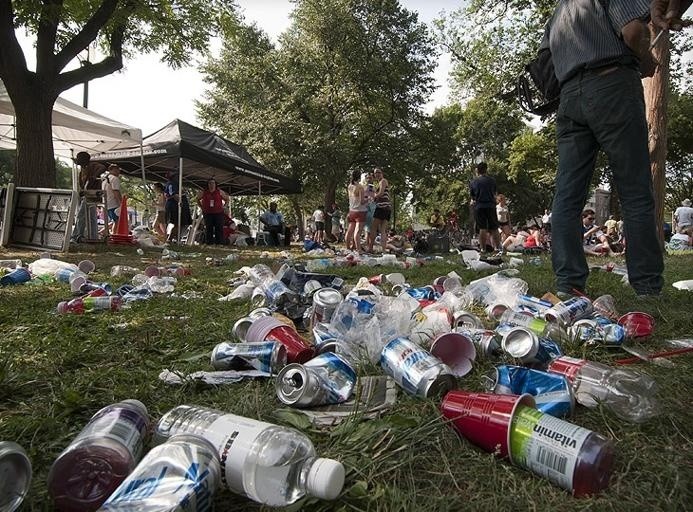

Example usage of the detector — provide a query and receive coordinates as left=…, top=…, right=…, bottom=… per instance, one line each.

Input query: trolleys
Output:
left=75, top=177, right=108, bottom=246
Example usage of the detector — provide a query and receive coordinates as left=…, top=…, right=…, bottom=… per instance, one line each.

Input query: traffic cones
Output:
left=108, top=193, right=135, bottom=246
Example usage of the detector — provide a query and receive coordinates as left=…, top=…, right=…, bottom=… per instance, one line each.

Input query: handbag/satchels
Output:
left=517, top=48, right=559, bottom=115
left=239, top=224, right=250, bottom=234
left=85, top=177, right=102, bottom=190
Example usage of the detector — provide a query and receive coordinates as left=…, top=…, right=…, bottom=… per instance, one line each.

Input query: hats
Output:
left=681, top=198, right=692, bottom=207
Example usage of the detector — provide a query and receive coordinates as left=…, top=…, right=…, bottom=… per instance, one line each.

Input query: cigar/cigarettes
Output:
left=646, top=29, right=664, bottom=51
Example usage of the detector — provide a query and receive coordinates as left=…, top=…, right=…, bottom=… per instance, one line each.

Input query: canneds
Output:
left=99, top=432, right=221, bottom=511
left=211, top=274, right=655, bottom=419
left=0, top=441, right=32, bottom=512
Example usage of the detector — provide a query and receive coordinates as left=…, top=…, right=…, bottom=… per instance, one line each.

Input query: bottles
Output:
left=57, top=294, right=123, bottom=314
left=509, top=404, right=616, bottom=501
left=304, top=259, right=340, bottom=271
left=606, top=263, right=629, bottom=276
left=399, top=261, right=414, bottom=269
left=153, top=405, right=346, bottom=507
left=247, top=262, right=287, bottom=301
left=546, top=354, right=659, bottom=424
left=54, top=267, right=90, bottom=284
left=46, top=399, right=149, bottom=511
left=499, top=310, right=569, bottom=344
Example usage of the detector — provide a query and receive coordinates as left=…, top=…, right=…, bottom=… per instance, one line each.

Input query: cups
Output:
left=0, top=267, right=31, bottom=288
left=78, top=260, right=95, bottom=274
left=429, top=334, right=477, bottom=376
left=438, top=390, right=535, bottom=462
left=591, top=294, right=619, bottom=321
left=244, top=317, right=312, bottom=363
left=618, top=311, right=656, bottom=342
left=176, top=267, right=190, bottom=277
left=144, top=265, right=161, bottom=277
left=434, top=276, right=462, bottom=292
left=370, top=274, right=385, bottom=285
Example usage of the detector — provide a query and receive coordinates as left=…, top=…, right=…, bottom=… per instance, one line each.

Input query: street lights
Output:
left=78, top=60, right=93, bottom=109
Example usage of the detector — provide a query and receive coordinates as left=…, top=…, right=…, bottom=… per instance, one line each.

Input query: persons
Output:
left=72, top=150, right=106, bottom=243
left=100, top=164, right=692, bottom=252
left=538, top=0, right=659, bottom=301
left=469, top=160, right=500, bottom=252
left=650, top=1, right=692, bottom=35
